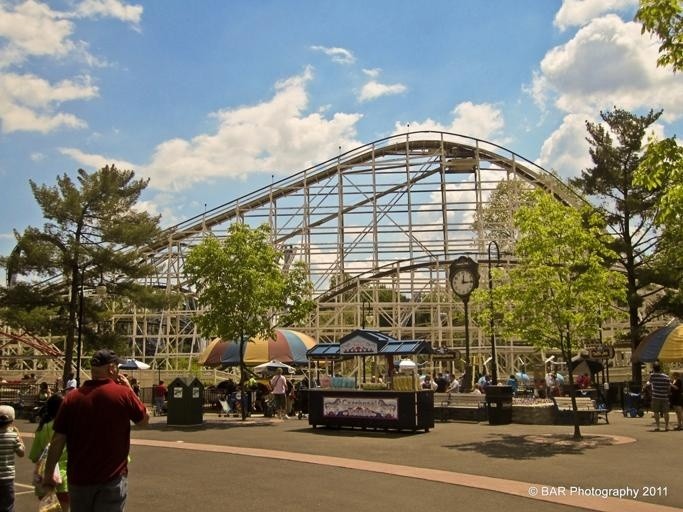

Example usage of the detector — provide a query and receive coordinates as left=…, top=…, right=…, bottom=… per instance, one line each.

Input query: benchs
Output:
left=549, top=395, right=609, bottom=425
left=433, top=392, right=488, bottom=423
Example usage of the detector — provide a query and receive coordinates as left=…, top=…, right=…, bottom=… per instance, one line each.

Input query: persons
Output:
left=38, top=348, right=149, bottom=511
left=37, top=382, right=53, bottom=407
left=29, top=392, right=73, bottom=511
left=0, top=403, right=26, bottom=511
left=153, top=378, right=168, bottom=414
left=221, top=367, right=317, bottom=420
left=130, top=378, right=141, bottom=398
left=668, top=371, right=682, bottom=432
left=641, top=359, right=671, bottom=432
left=393, top=355, right=592, bottom=398
left=62, top=372, right=76, bottom=395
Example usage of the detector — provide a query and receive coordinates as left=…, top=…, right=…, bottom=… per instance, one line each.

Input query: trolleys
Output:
left=621, top=379, right=643, bottom=418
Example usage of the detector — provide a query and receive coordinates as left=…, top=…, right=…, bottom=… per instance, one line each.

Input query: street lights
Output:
left=76, top=259, right=107, bottom=389
left=486, top=239, right=506, bottom=385
left=362, top=298, right=375, bottom=385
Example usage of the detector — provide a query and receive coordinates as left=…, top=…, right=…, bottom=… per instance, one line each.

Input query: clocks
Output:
left=451, top=271, right=474, bottom=295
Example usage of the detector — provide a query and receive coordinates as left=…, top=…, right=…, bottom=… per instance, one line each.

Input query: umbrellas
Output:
left=198, top=328, right=334, bottom=368
left=251, top=356, right=297, bottom=376
left=117, top=357, right=150, bottom=379
left=628, top=319, right=682, bottom=362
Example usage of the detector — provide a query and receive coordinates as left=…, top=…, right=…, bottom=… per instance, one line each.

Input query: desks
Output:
left=307, top=387, right=434, bottom=433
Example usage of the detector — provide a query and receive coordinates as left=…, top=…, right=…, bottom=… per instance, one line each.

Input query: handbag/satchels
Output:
left=32, top=441, right=63, bottom=486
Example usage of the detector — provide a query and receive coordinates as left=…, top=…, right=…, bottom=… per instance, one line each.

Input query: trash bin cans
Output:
left=486, top=385, right=512, bottom=425
left=166, top=375, right=206, bottom=427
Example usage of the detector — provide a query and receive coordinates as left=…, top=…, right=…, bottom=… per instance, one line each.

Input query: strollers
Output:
left=216, top=394, right=239, bottom=418
left=295, top=392, right=309, bottom=420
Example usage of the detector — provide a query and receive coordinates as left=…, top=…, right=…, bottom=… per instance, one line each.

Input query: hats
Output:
left=0, top=405, right=15, bottom=422
left=90, top=349, right=127, bottom=367
